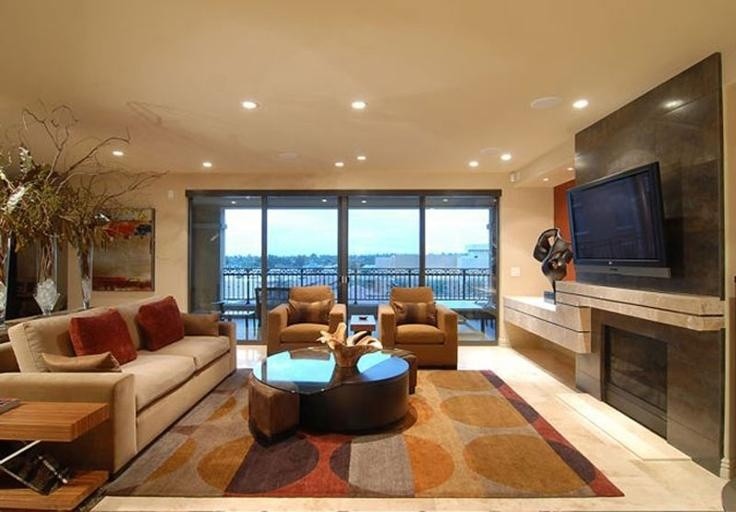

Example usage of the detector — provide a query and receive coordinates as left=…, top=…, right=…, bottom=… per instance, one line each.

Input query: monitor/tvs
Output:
left=566, top=162, right=672, bottom=278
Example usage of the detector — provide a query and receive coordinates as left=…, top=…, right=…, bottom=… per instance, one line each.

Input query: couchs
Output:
left=6, top=293, right=237, bottom=476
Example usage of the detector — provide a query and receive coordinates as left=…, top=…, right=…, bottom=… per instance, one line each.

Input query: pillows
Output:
left=181, top=311, right=221, bottom=336
left=287, top=298, right=332, bottom=324
left=134, top=295, right=184, bottom=351
left=392, top=301, right=438, bottom=327
left=39, top=349, right=123, bottom=374
left=68, top=303, right=137, bottom=364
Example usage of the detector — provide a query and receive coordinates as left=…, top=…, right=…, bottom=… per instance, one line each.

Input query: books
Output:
left=0, top=396, right=23, bottom=418
left=0, top=440, right=76, bottom=496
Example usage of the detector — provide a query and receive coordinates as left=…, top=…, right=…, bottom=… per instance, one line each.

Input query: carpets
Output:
left=99, top=367, right=625, bottom=499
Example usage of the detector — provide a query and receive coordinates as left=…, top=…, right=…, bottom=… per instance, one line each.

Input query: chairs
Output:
left=269, top=284, right=347, bottom=351
left=378, top=286, right=457, bottom=368
left=254, top=288, right=289, bottom=327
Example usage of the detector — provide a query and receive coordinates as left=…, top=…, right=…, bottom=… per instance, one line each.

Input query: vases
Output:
left=32, top=234, right=61, bottom=314
left=81, top=239, right=93, bottom=310
left=0, top=231, right=12, bottom=327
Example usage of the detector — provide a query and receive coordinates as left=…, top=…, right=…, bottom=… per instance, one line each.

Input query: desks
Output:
left=0, top=398, right=112, bottom=511
left=351, top=314, right=376, bottom=337
left=215, top=299, right=256, bottom=336
